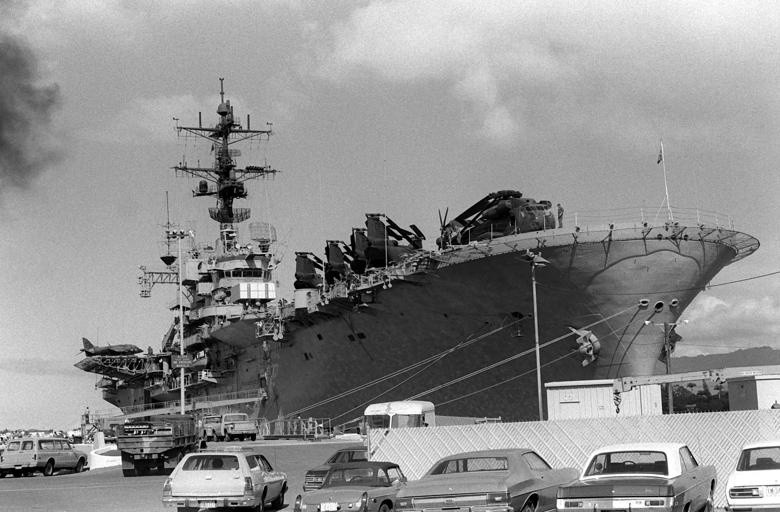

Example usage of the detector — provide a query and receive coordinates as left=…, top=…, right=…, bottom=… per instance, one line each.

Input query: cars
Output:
left=302, top=445, right=369, bottom=491
left=161, top=450, right=288, bottom=511
left=293, top=460, right=409, bottom=511
left=200, top=413, right=260, bottom=440
left=392, top=448, right=580, bottom=510
left=555, top=441, right=717, bottom=511
left=91, top=429, right=118, bottom=444
left=725, top=440, right=780, bottom=512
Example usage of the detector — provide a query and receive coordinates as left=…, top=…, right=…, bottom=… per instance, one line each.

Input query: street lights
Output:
left=641, top=317, right=692, bottom=416
left=522, top=247, right=553, bottom=421
left=165, top=228, right=198, bottom=416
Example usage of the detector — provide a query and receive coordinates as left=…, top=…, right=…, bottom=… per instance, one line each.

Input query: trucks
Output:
left=116, top=412, right=198, bottom=477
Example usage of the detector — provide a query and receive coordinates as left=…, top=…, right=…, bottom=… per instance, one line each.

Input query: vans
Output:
left=357, top=398, right=436, bottom=446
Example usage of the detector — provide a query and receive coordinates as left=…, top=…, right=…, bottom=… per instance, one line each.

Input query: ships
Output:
left=75, top=74, right=760, bottom=440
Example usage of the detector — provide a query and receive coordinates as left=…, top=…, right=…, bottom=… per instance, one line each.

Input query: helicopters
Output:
left=295, top=186, right=555, bottom=290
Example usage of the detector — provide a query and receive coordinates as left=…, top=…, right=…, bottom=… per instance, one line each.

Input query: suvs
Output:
left=0, top=437, right=89, bottom=476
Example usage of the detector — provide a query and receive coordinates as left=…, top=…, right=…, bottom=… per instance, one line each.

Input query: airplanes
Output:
left=78, top=335, right=143, bottom=358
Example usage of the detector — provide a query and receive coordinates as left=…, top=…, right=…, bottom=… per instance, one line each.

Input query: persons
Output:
left=85, top=406, right=90, bottom=424
left=543, top=206, right=552, bottom=228
left=556, top=203, right=564, bottom=228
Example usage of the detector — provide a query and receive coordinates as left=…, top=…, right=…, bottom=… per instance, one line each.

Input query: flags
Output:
left=656, top=145, right=664, bottom=164
